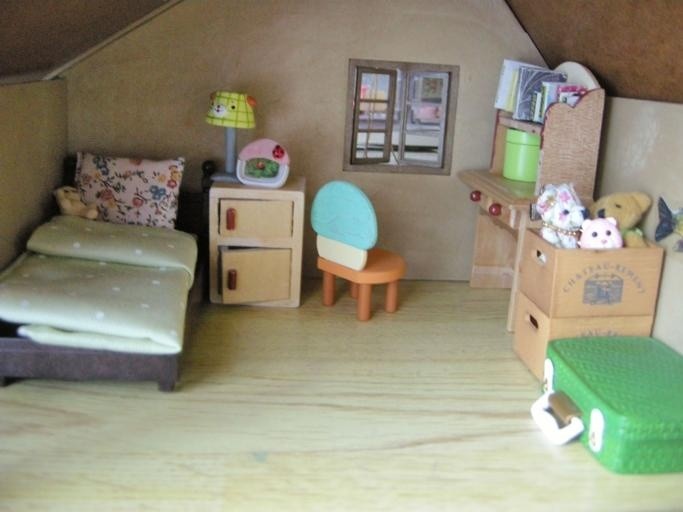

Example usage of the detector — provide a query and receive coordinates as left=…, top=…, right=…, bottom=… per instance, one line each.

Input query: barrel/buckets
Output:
left=503, top=128, right=540, bottom=184
